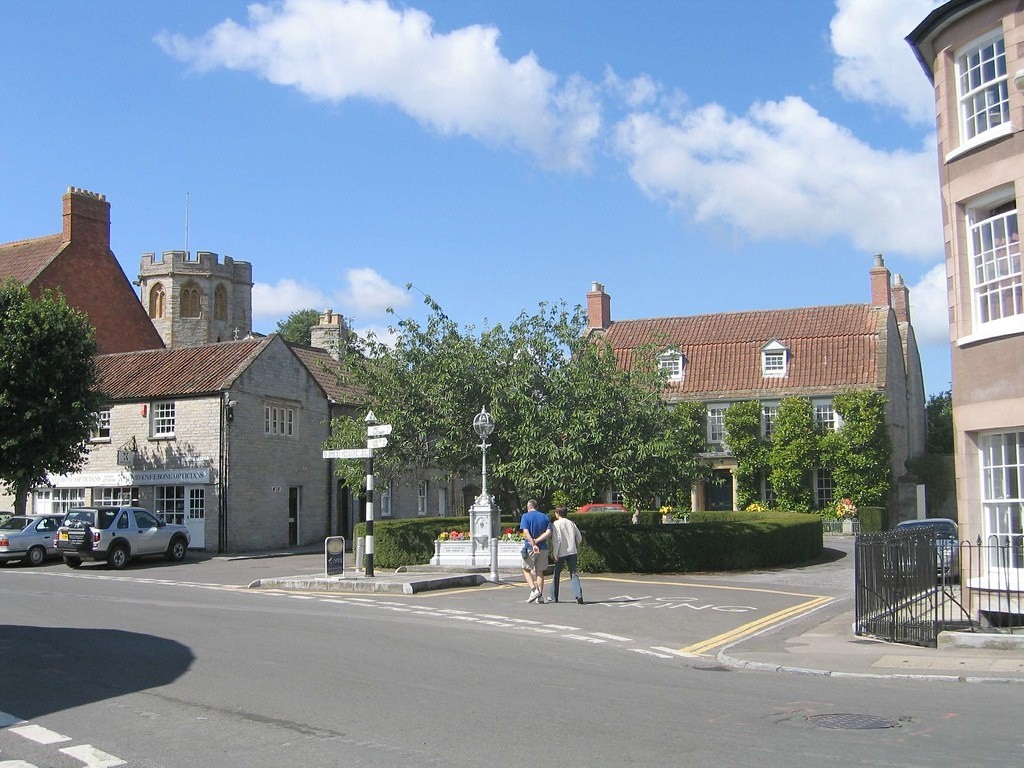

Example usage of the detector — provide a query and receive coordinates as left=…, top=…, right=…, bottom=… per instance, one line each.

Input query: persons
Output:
left=547, top=504, right=584, bottom=603
left=519, top=499, right=554, bottom=603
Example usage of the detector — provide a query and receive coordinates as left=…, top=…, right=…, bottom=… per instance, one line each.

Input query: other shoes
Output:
left=577, top=596, right=583, bottom=604
left=547, top=596, right=557, bottom=602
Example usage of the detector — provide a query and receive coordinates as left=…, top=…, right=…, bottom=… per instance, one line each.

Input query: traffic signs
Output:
left=322, top=449, right=372, bottom=459
left=366, top=425, right=393, bottom=436
left=367, top=437, right=389, bottom=449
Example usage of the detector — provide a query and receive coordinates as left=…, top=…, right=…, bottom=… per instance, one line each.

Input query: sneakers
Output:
left=536, top=596, right=544, bottom=603
left=527, top=589, right=540, bottom=603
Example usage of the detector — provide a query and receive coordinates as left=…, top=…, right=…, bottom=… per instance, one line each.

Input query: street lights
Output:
left=468, top=405, right=502, bottom=539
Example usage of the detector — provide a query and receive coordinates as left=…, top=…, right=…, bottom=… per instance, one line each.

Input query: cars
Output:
left=0, top=513, right=83, bottom=567
left=578, top=504, right=628, bottom=514
left=882, top=518, right=960, bottom=582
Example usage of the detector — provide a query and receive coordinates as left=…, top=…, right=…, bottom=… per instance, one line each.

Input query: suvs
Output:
left=52, top=505, right=192, bottom=570
